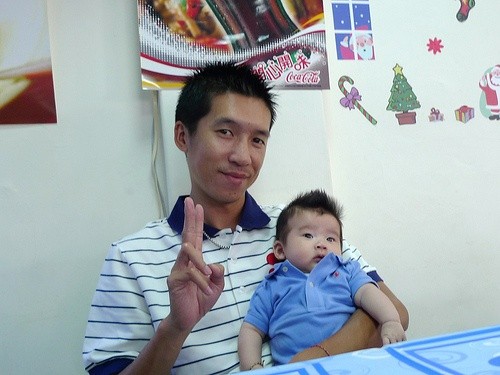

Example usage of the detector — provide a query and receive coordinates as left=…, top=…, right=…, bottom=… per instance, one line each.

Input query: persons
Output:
left=81, top=59, right=409, bottom=375
left=238, top=188, right=407, bottom=371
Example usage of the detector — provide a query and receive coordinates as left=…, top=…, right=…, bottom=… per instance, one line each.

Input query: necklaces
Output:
left=203, top=231, right=231, bottom=248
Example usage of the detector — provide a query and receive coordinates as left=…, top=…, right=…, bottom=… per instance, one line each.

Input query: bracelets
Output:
left=310, top=344, right=331, bottom=356
left=247, top=360, right=265, bottom=370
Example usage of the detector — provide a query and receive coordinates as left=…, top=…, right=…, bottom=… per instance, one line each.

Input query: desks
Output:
left=220, top=326, right=500, bottom=375
